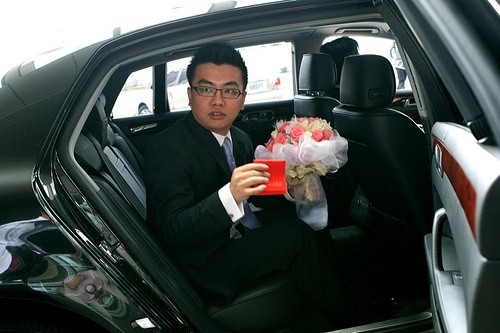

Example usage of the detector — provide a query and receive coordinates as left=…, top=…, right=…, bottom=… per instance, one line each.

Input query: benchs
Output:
left=70, top=93, right=306, bottom=333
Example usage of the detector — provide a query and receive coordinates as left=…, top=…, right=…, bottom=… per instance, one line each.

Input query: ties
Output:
left=223, top=137, right=264, bottom=230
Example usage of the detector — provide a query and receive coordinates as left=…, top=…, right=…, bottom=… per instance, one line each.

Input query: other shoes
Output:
left=346, top=294, right=401, bottom=326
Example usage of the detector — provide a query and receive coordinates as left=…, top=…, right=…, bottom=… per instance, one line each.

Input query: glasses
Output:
left=190, top=86, right=245, bottom=99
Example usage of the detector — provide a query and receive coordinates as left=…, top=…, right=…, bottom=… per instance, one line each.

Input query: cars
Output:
left=0, top=1, right=500, bottom=332
left=134, top=64, right=283, bottom=115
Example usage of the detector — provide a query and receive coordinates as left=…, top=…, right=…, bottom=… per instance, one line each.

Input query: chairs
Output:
left=332, top=54, right=434, bottom=257
left=295, top=52, right=348, bottom=223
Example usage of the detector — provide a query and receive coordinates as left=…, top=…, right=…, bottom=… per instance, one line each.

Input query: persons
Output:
left=320, top=37, right=358, bottom=100
left=144, top=41, right=400, bottom=327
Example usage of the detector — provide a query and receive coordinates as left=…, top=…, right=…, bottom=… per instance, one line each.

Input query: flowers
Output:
left=253, top=114, right=350, bottom=230
